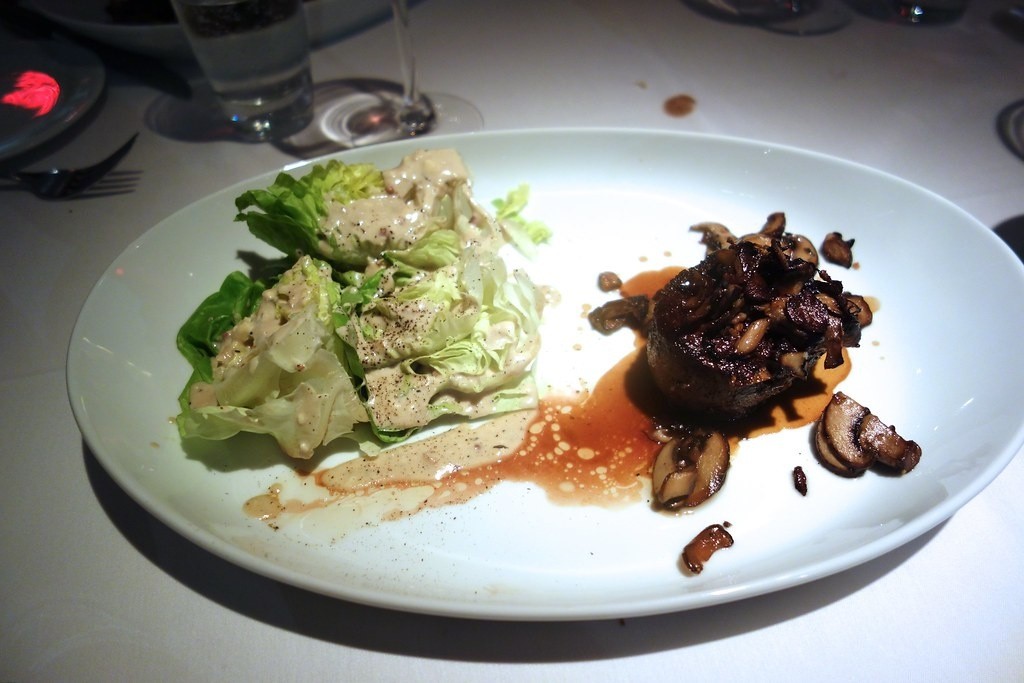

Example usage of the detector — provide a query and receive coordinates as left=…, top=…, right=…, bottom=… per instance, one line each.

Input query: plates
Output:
left=66, top=127, right=1024, bottom=622
left=0, top=37, right=106, bottom=158
left=15, top=0, right=418, bottom=56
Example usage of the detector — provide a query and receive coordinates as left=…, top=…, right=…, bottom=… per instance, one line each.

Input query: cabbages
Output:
left=174, top=149, right=552, bottom=462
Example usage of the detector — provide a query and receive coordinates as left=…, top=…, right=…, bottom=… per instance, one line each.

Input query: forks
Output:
left=0, top=133, right=138, bottom=200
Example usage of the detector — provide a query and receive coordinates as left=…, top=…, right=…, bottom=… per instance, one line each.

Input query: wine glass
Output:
left=320, top=0, right=484, bottom=147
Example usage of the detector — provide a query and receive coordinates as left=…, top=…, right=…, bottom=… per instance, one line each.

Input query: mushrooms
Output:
left=587, top=212, right=922, bottom=575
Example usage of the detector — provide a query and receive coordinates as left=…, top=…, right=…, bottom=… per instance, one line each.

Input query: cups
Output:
left=168, top=1, right=314, bottom=142
